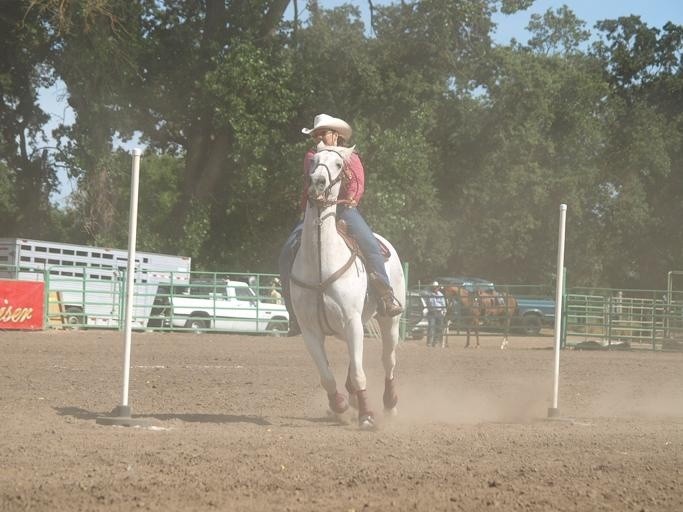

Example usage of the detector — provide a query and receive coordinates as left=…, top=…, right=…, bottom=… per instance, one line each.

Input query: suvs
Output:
left=365, top=292, right=428, bottom=338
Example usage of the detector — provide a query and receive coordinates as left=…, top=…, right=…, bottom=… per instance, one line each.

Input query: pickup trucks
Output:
left=161, top=279, right=290, bottom=337
left=423, top=275, right=556, bottom=334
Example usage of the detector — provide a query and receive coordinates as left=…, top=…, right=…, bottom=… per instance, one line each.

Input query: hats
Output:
left=302, top=113, right=352, bottom=140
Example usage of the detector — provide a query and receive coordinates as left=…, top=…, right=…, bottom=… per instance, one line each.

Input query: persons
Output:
left=265, top=282, right=281, bottom=304
left=421, top=281, right=447, bottom=347
left=279, top=114, right=402, bottom=337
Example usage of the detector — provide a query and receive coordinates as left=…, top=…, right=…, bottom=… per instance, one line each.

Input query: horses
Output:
left=289, top=140, right=406, bottom=431
left=443, top=283, right=520, bottom=351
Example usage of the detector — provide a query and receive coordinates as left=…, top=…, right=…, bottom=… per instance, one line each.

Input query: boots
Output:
left=381, top=289, right=402, bottom=317
